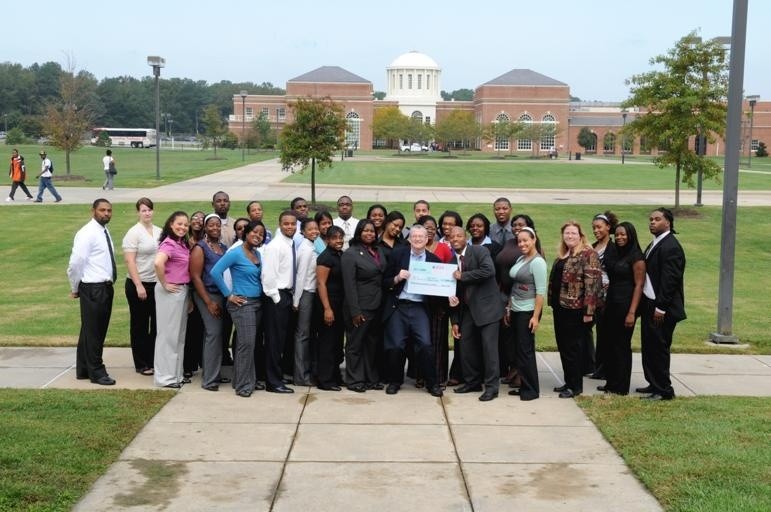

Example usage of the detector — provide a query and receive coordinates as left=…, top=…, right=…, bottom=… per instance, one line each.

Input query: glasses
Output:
left=192, top=215, right=205, bottom=221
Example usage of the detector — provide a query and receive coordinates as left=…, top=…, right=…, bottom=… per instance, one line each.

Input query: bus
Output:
left=90, top=126, right=156, bottom=149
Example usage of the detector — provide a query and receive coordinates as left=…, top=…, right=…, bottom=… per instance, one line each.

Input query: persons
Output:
left=548, top=206, right=689, bottom=402
left=330, top=196, right=550, bottom=401
left=101, top=150, right=115, bottom=190
left=123, top=189, right=348, bottom=397
left=33, top=150, right=62, bottom=202
left=5, top=148, right=33, bottom=201
left=68, top=197, right=118, bottom=385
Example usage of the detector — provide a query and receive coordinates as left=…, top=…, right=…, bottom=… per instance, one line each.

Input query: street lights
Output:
left=620, top=113, right=626, bottom=165
left=146, top=54, right=165, bottom=180
left=679, top=35, right=731, bottom=207
left=240, top=90, right=247, bottom=161
left=747, top=100, right=756, bottom=167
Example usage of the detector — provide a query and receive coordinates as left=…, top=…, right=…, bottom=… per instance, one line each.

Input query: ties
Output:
left=646, top=242, right=656, bottom=259
left=291, top=240, right=299, bottom=293
left=102, top=227, right=120, bottom=284
left=460, top=255, right=470, bottom=306
left=343, top=222, right=352, bottom=252
left=501, top=229, right=509, bottom=247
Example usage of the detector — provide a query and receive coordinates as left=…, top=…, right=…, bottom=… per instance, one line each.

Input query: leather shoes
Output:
left=559, top=386, right=584, bottom=398
left=166, top=370, right=193, bottom=389
left=254, top=383, right=265, bottom=391
left=282, top=379, right=294, bottom=385
left=386, top=383, right=401, bottom=394
left=267, top=385, right=294, bottom=393
left=508, top=387, right=523, bottom=395
left=453, top=382, right=485, bottom=393
left=76, top=371, right=92, bottom=380
left=206, top=385, right=219, bottom=391
left=639, top=389, right=676, bottom=402
left=499, top=368, right=519, bottom=384
left=427, top=384, right=442, bottom=396
left=329, top=385, right=341, bottom=391
left=353, top=386, right=366, bottom=392
left=446, top=375, right=465, bottom=387
left=509, top=372, right=525, bottom=388
left=554, top=382, right=569, bottom=392
left=604, top=390, right=630, bottom=396
left=90, top=373, right=117, bottom=385
left=597, top=383, right=618, bottom=392
left=241, top=390, right=252, bottom=397
left=520, top=391, right=541, bottom=400
left=635, top=382, right=662, bottom=393
left=375, top=384, right=383, bottom=389
left=479, top=389, right=501, bottom=401
left=220, top=378, right=232, bottom=383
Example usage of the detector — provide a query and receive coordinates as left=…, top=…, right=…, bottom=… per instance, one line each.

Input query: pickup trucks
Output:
left=401, top=142, right=428, bottom=152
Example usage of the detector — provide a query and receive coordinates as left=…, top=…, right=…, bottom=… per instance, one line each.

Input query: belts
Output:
left=176, top=281, right=191, bottom=286
left=278, top=287, right=295, bottom=293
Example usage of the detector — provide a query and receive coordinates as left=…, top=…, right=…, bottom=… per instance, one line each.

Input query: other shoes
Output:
left=139, top=366, right=154, bottom=375
left=415, top=378, right=425, bottom=388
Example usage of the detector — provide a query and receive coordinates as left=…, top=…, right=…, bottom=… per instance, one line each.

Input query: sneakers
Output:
left=5, top=196, right=62, bottom=204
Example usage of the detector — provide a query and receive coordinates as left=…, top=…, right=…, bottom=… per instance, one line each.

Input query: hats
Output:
left=39, top=150, right=48, bottom=156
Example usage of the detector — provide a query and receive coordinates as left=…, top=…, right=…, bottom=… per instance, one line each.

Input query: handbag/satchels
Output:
left=108, top=160, right=117, bottom=176
